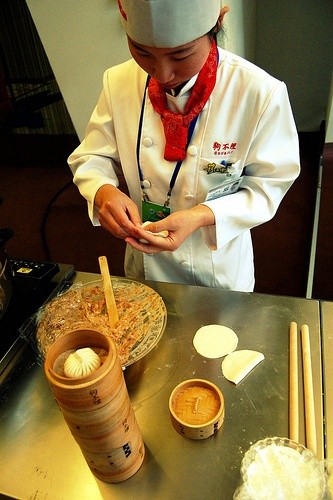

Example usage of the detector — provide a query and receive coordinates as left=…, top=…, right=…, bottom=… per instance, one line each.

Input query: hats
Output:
left=115, top=1, right=220, bottom=48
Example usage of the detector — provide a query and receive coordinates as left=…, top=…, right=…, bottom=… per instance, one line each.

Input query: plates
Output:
left=35, top=277, right=168, bottom=370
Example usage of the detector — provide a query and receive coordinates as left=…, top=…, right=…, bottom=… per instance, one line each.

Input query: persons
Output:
left=65, top=0, right=302, bottom=290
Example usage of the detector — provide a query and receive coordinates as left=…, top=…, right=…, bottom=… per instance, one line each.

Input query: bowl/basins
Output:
left=240, top=437, right=327, bottom=500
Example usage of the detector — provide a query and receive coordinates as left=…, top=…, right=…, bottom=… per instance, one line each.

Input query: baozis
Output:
left=62, top=346, right=101, bottom=379
left=137, top=221, right=168, bottom=244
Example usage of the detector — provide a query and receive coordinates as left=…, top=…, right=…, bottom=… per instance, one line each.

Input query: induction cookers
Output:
left=0, top=259, right=60, bottom=381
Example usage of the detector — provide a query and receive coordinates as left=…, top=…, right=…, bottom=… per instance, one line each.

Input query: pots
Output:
left=0, top=227, right=16, bottom=321
